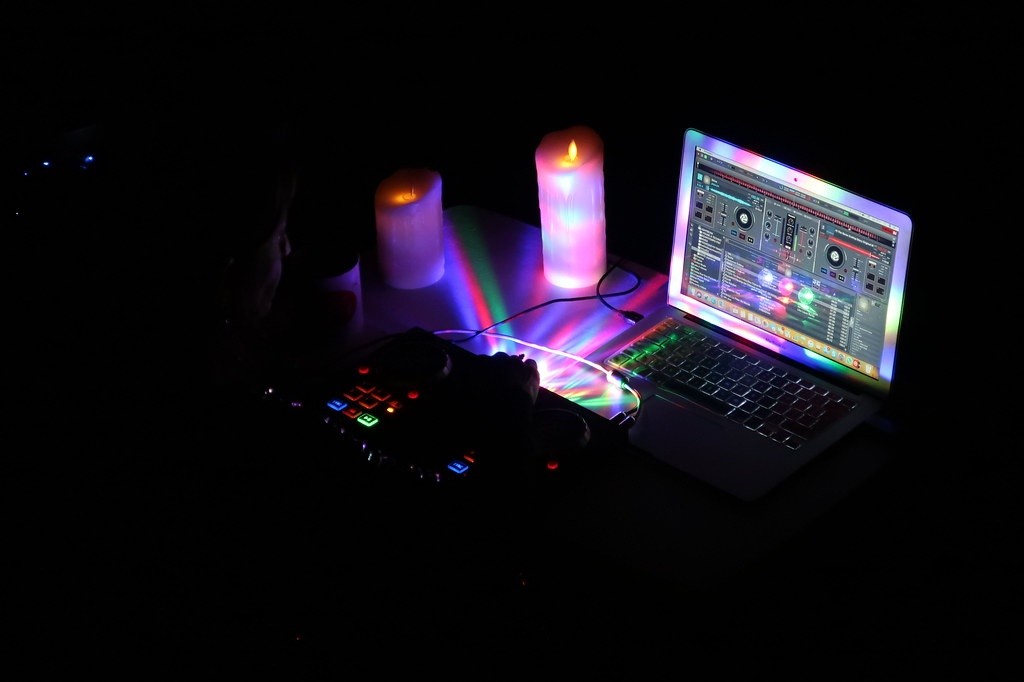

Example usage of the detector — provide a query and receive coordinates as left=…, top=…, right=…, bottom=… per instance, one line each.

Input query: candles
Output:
left=374, top=167, right=444, bottom=290
left=535, top=129, right=608, bottom=288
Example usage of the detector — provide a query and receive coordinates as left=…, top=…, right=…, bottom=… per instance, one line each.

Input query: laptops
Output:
left=540, top=126, right=914, bottom=507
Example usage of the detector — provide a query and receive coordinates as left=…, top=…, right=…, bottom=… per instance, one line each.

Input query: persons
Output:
left=180, top=141, right=540, bottom=445
